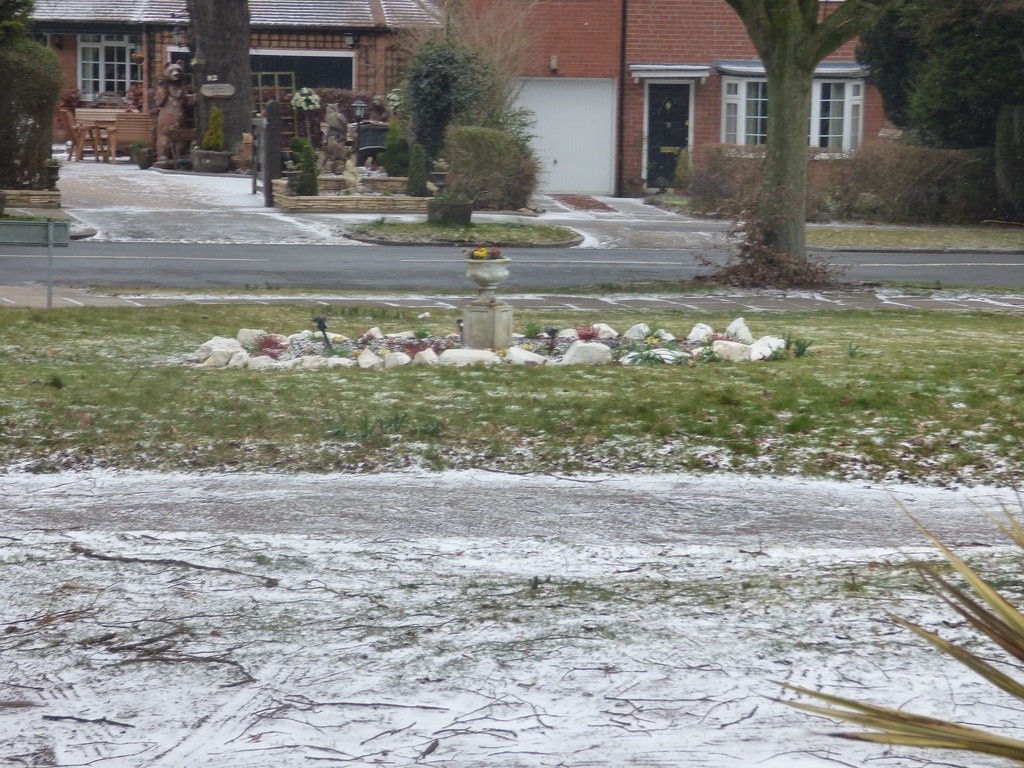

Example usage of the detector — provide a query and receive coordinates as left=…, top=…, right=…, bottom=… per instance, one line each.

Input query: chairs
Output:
left=105, top=113, right=152, bottom=163
left=60, top=110, right=108, bottom=162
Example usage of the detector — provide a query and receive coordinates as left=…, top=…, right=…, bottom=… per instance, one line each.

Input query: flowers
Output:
left=462, top=246, right=501, bottom=260
left=387, top=88, right=403, bottom=112
left=290, top=87, right=322, bottom=112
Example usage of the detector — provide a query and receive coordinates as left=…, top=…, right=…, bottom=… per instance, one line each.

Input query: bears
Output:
left=154, top=60, right=195, bottom=163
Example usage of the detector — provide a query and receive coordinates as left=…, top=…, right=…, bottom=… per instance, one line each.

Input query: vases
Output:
left=465, top=256, right=512, bottom=305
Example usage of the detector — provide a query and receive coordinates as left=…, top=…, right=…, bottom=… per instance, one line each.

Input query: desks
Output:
left=95, top=120, right=116, bottom=126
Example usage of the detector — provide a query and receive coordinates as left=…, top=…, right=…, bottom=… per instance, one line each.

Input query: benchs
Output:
left=75, top=108, right=140, bottom=157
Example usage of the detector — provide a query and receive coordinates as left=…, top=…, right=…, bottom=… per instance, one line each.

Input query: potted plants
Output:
left=190, top=107, right=233, bottom=172
left=132, top=49, right=145, bottom=64
left=191, top=46, right=206, bottom=72
left=428, top=188, right=479, bottom=224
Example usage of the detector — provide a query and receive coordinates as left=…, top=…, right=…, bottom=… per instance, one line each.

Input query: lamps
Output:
left=351, top=100, right=367, bottom=122
left=171, top=26, right=190, bottom=48
left=345, top=32, right=360, bottom=45
